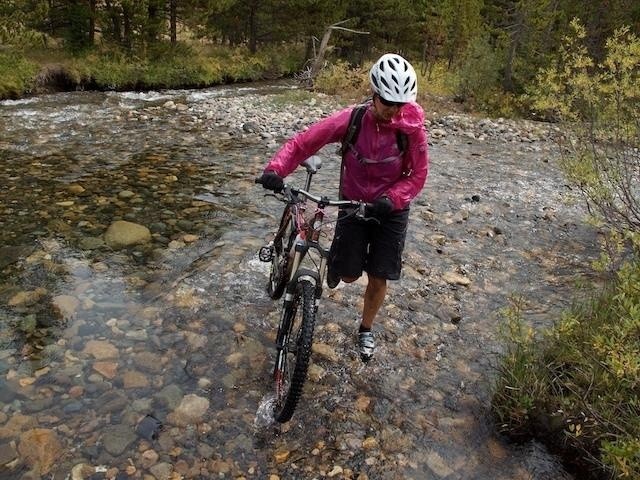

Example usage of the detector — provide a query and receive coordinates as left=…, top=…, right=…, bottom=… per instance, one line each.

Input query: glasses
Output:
left=379, top=98, right=407, bottom=107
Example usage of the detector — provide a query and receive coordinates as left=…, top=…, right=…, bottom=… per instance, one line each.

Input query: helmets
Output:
left=369, top=53, right=418, bottom=103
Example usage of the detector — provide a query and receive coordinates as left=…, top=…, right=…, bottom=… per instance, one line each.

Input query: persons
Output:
left=263, top=53, right=428, bottom=360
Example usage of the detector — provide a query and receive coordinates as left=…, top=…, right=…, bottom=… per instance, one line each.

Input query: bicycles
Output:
left=258, top=155, right=381, bottom=423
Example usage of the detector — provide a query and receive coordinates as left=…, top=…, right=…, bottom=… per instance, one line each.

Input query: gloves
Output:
left=376, top=197, right=393, bottom=214
left=261, top=173, right=283, bottom=194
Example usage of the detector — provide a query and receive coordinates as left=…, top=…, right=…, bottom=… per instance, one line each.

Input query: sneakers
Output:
left=327, top=262, right=340, bottom=289
left=357, top=327, right=375, bottom=362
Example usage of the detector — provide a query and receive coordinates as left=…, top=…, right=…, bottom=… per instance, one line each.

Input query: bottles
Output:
left=289, top=231, right=307, bottom=259
